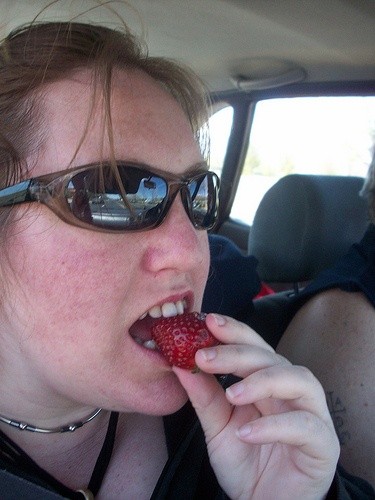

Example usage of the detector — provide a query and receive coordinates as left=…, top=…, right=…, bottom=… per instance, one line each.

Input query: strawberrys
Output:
left=151, top=311, right=223, bottom=373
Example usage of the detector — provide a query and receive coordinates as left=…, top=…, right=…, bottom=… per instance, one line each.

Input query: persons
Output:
left=274, top=143, right=374, bottom=499
left=1, top=0, right=341, bottom=500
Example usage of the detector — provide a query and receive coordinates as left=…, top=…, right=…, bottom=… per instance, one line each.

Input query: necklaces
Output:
left=1, top=407, right=103, bottom=433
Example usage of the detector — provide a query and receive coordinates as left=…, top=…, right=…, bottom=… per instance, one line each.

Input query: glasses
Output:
left=1, top=160, right=223, bottom=234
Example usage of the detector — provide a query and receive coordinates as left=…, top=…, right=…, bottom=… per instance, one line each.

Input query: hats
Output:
left=358, top=149, right=375, bottom=199
